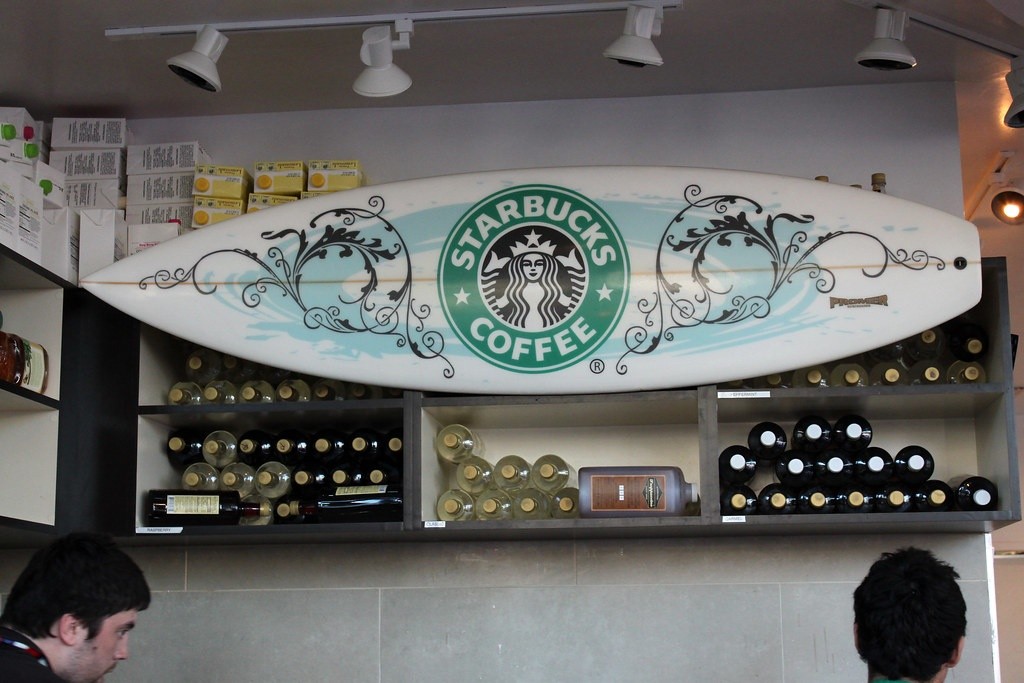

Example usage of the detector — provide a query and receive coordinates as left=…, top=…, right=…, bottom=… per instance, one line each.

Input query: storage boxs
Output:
left=1, top=105, right=368, bottom=287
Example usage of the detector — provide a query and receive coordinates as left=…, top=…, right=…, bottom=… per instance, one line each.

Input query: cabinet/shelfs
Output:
left=1, top=245, right=1023, bottom=549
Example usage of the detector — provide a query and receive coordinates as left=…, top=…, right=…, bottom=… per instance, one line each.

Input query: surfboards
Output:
left=74, top=161, right=986, bottom=400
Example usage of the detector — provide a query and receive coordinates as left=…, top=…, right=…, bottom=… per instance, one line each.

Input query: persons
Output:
left=0, top=531, right=151, bottom=682
left=852, top=545, right=967, bottom=683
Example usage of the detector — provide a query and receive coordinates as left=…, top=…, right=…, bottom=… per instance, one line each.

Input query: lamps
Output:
left=1002, top=55, right=1024, bottom=130
left=989, top=173, right=1024, bottom=225
left=602, top=4, right=667, bottom=68
left=164, top=25, right=231, bottom=95
left=351, top=19, right=417, bottom=99
left=853, top=7, right=918, bottom=71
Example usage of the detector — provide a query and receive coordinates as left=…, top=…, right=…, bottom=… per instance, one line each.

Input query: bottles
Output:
left=435, top=423, right=701, bottom=520
left=717, top=414, right=999, bottom=512
left=144, top=353, right=401, bottom=527
left=725, top=327, right=987, bottom=391
left=1, top=330, right=51, bottom=397
